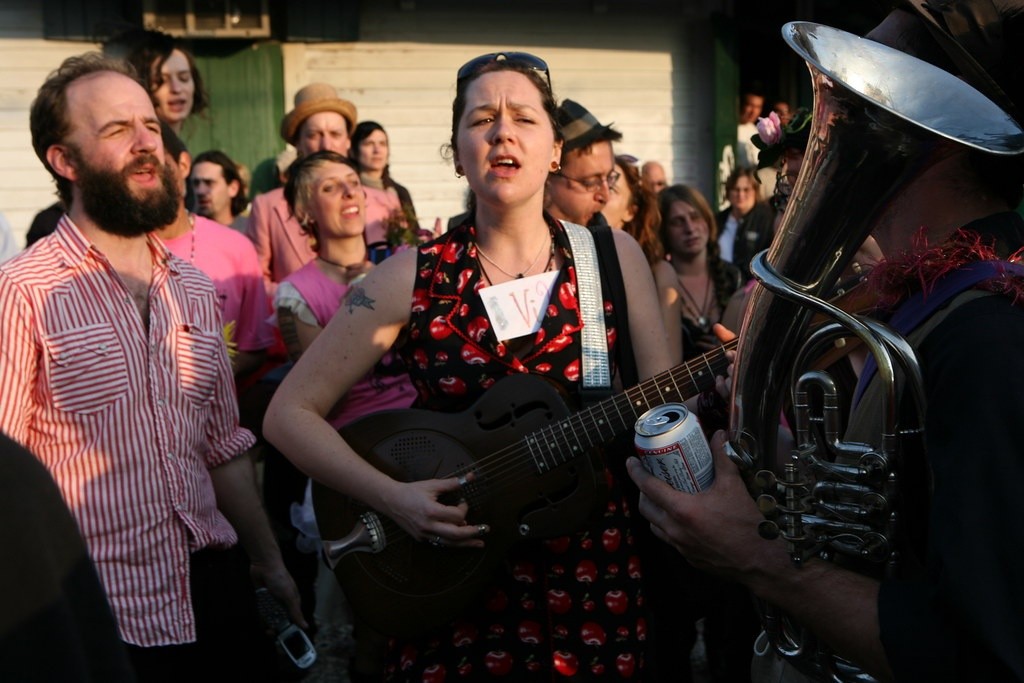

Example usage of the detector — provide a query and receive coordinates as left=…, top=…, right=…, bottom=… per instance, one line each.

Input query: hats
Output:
left=557, top=99, right=622, bottom=150
left=866, top=9, right=994, bottom=98
left=281, top=83, right=358, bottom=145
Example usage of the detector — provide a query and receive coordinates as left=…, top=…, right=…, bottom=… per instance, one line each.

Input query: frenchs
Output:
left=724, top=21, right=1024, bottom=683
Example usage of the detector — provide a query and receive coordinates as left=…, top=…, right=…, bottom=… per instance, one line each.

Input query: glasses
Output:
left=456, top=52, right=551, bottom=96
left=551, top=170, right=620, bottom=193
left=776, top=173, right=797, bottom=195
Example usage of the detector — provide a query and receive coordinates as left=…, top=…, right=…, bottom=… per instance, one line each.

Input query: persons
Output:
left=21, top=0, right=1022, bottom=683
left=0, top=431, right=137, bottom=683
left=2, top=43, right=316, bottom=683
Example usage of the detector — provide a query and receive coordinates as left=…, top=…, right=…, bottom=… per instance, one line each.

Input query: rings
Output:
left=476, top=524, right=486, bottom=535
left=459, top=475, right=468, bottom=488
left=430, top=534, right=440, bottom=546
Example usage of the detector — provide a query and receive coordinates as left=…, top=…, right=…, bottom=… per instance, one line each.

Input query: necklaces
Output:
left=468, top=234, right=550, bottom=282
left=182, top=205, right=198, bottom=265
left=316, top=251, right=369, bottom=271
left=675, top=269, right=713, bottom=326
left=478, top=246, right=554, bottom=291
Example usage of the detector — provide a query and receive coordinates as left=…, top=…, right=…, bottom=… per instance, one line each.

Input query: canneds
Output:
left=633, top=402, right=714, bottom=494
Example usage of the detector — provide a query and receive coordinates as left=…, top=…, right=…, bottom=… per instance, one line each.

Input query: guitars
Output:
left=311, top=273, right=901, bottom=597
